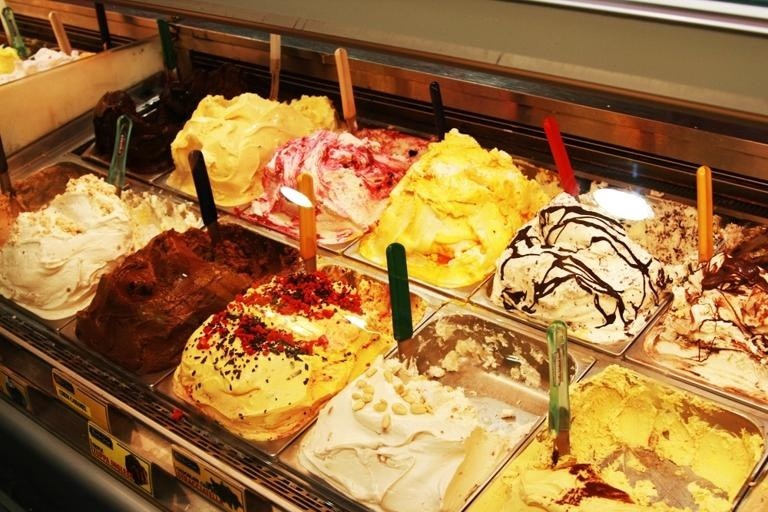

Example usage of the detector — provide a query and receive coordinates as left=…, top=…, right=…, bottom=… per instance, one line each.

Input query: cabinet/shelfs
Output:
left=0, top=0, right=768, bottom=512
